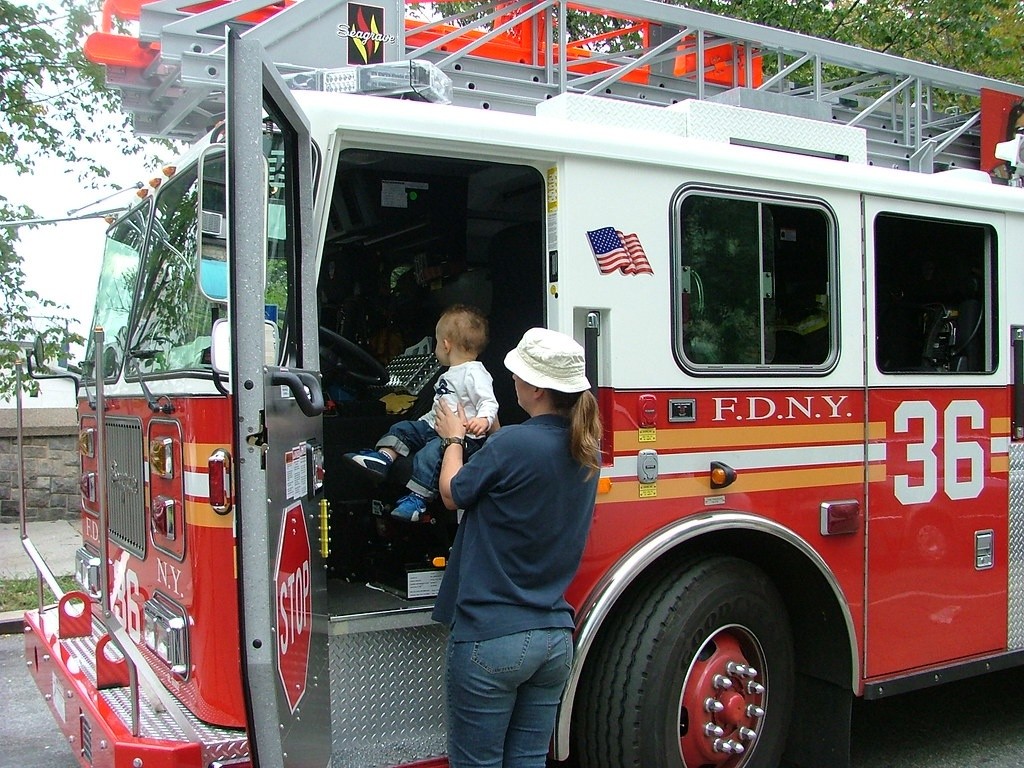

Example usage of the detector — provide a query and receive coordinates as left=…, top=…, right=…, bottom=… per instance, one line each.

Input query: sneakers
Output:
left=341, top=447, right=391, bottom=480
left=390, top=492, right=429, bottom=525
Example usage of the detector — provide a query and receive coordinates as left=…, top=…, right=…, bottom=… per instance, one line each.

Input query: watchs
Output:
left=440, top=437, right=465, bottom=459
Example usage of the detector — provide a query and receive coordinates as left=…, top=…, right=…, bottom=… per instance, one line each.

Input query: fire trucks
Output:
left=20, top=0, right=1024, bottom=767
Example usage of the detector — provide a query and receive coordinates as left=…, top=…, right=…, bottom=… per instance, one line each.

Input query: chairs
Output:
left=382, top=219, right=542, bottom=514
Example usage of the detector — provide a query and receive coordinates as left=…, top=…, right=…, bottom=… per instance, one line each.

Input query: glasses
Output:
left=1014, top=127, right=1024, bottom=134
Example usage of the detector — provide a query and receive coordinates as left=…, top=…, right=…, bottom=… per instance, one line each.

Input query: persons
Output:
left=342, top=305, right=499, bottom=521
left=431, top=328, right=601, bottom=768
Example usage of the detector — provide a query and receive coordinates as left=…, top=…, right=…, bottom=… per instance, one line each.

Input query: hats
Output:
left=503, top=327, right=592, bottom=392
